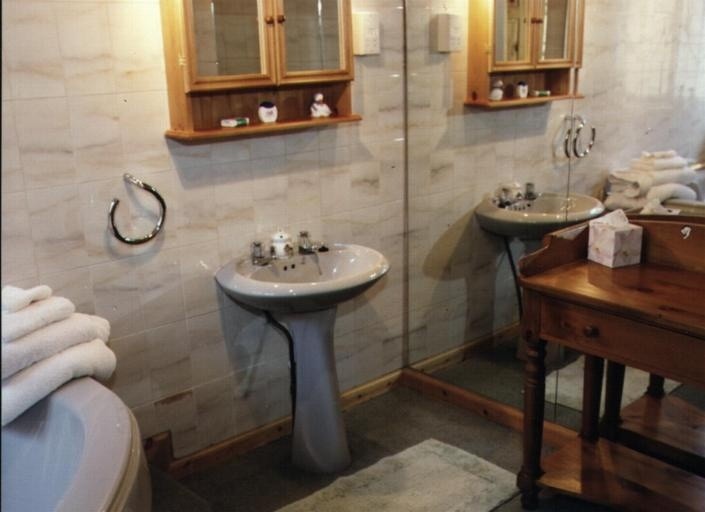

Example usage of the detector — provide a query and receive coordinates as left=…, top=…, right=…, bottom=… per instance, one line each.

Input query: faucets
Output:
left=298, top=230, right=319, bottom=254
left=252, top=241, right=277, bottom=265
left=525, top=182, right=545, bottom=201
left=498, top=184, right=512, bottom=208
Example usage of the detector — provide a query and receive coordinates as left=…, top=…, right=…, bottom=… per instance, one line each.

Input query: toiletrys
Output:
left=310, top=94, right=330, bottom=119
left=515, top=80, right=529, bottom=99
left=490, top=80, right=504, bottom=101
left=259, top=102, right=279, bottom=123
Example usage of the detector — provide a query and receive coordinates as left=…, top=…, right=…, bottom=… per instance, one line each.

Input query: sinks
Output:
left=475, top=190, right=606, bottom=237
left=216, top=242, right=392, bottom=314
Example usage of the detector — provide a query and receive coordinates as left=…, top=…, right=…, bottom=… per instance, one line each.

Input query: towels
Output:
left=605, top=149, right=705, bottom=218
left=0, top=282, right=117, bottom=430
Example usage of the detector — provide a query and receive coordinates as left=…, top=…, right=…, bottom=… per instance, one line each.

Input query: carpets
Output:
left=273, top=437, right=522, bottom=510
left=521, top=354, right=683, bottom=413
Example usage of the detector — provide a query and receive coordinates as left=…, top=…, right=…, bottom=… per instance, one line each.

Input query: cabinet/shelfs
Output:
left=467, top=0, right=586, bottom=110
left=155, top=1, right=362, bottom=144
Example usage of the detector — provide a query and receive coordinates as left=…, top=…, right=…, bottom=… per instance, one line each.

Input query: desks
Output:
left=513, top=223, right=704, bottom=511
left=600, top=215, right=705, bottom=475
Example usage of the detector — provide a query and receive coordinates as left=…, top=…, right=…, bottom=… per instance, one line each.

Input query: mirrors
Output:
left=495, top=1, right=530, bottom=65
left=544, top=1, right=570, bottom=64
left=402, top=1, right=704, bottom=437
left=191, top=0, right=262, bottom=81
left=285, top=1, right=344, bottom=76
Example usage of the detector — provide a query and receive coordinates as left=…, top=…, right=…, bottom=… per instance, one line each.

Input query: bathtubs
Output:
left=1, top=374, right=152, bottom=512
left=663, top=169, right=705, bottom=209
